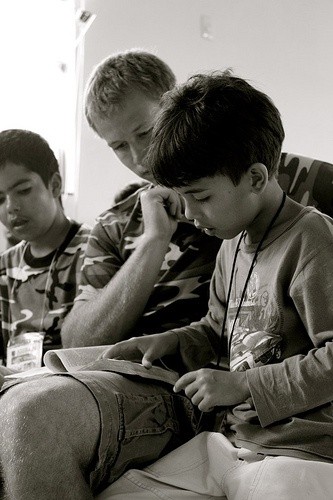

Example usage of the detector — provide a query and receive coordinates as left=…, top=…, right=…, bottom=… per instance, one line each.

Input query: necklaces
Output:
left=217, top=191, right=286, bottom=370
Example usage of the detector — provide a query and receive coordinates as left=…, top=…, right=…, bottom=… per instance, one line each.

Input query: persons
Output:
left=92, top=66, right=333, bottom=500
left=0, top=50, right=333, bottom=499
left=0, top=128, right=93, bottom=398
